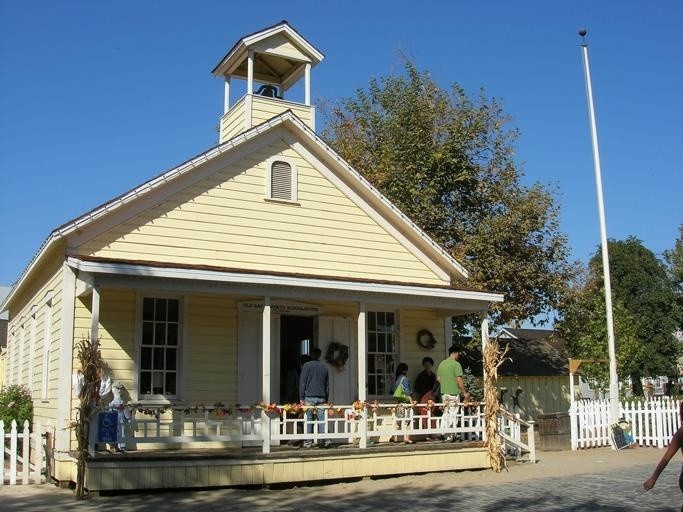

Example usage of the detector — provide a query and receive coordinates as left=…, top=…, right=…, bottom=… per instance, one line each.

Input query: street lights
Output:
left=577, top=28, right=621, bottom=449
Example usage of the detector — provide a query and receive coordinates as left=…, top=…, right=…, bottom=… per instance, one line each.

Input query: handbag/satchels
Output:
left=393, top=383, right=410, bottom=403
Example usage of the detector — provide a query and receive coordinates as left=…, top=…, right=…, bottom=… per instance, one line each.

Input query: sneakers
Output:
left=404, top=433, right=456, bottom=444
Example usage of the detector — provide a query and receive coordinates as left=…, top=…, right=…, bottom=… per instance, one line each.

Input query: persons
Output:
left=412, top=355, right=440, bottom=441
left=106, top=382, right=133, bottom=451
left=298, top=347, right=329, bottom=450
left=389, top=363, right=416, bottom=444
left=435, top=346, right=470, bottom=443
left=284, top=360, right=303, bottom=445
left=641, top=400, right=682, bottom=512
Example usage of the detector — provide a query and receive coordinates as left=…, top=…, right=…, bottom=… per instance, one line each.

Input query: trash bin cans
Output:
left=537, top=411, right=572, bottom=451
